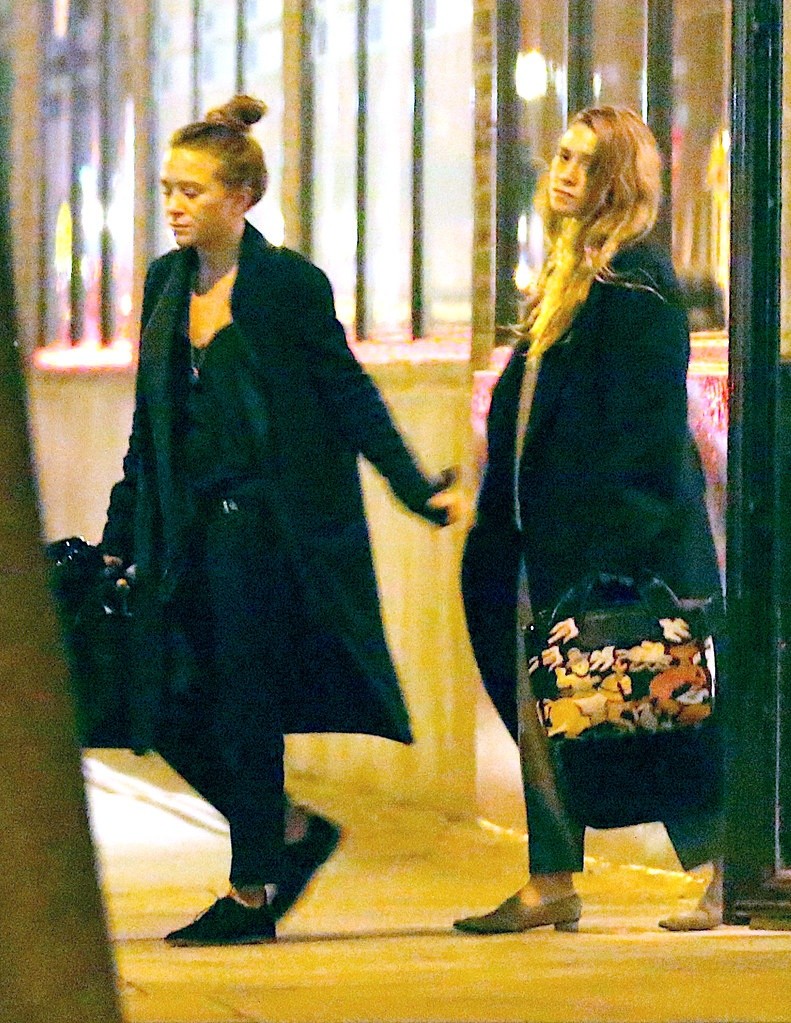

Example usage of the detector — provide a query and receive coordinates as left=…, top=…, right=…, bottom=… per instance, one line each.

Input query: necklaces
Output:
left=186, top=276, right=234, bottom=386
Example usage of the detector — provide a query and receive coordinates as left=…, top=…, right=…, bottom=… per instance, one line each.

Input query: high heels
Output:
left=657, top=906, right=719, bottom=931
left=452, top=894, right=583, bottom=934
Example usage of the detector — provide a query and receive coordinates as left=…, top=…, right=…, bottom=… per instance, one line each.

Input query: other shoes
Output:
left=270, top=808, right=341, bottom=923
left=165, top=896, right=277, bottom=945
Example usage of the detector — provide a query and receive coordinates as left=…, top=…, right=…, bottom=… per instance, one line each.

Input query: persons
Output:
left=453, top=106, right=728, bottom=933
left=93, top=94, right=459, bottom=944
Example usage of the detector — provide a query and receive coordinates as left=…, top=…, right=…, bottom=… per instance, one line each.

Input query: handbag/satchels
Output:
left=522, top=570, right=731, bottom=828
left=48, top=534, right=131, bottom=754
